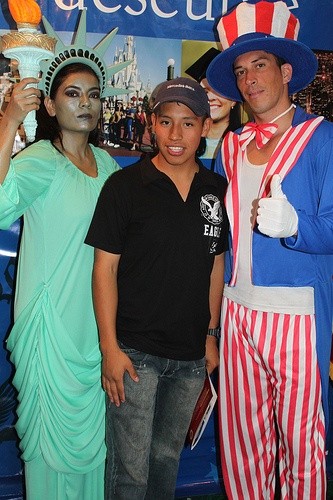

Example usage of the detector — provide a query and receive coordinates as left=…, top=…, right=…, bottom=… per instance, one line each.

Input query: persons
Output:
left=0, top=8, right=123, bottom=500
left=185, top=47, right=245, bottom=159
left=205, top=1, right=333, bottom=500
left=83, top=77, right=230, bottom=500
left=102, top=100, right=156, bottom=153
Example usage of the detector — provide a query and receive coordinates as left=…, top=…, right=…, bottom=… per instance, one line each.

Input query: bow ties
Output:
left=240, top=103, right=296, bottom=152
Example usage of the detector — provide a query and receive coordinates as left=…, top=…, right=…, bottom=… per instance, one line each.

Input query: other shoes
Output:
left=104, top=140, right=138, bottom=150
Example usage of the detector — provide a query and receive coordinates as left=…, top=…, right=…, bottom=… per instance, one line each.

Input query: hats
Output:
left=37, top=7, right=135, bottom=100
left=185, top=46, right=224, bottom=83
left=153, top=78, right=210, bottom=117
left=207, top=1, right=318, bottom=102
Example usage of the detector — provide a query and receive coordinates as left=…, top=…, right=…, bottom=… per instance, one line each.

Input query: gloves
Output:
left=258, top=173, right=299, bottom=237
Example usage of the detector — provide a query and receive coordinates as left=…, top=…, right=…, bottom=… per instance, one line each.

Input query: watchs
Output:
left=207, top=327, right=221, bottom=338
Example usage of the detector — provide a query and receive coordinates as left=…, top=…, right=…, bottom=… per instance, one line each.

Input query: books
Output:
left=187, top=376, right=218, bottom=450
